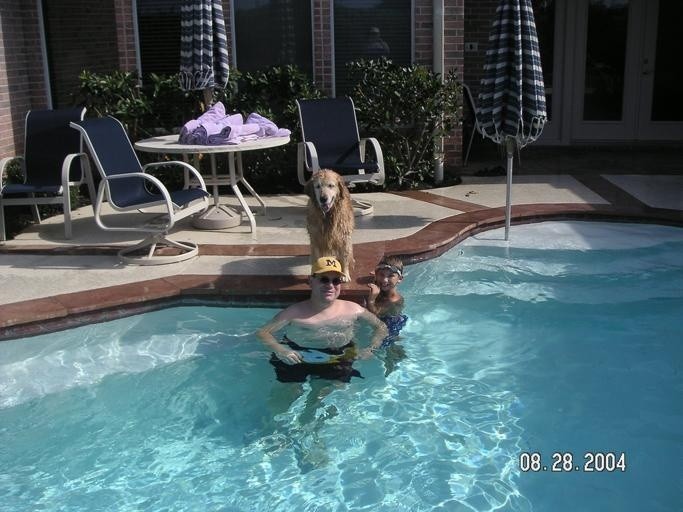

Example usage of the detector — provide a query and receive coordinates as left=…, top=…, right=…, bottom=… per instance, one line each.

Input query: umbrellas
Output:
left=472, top=0, right=550, bottom=242
left=174, top=0, right=233, bottom=118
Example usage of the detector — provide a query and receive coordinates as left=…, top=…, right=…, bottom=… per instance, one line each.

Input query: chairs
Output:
left=68, top=115, right=211, bottom=264
left=294, top=96, right=386, bottom=218
left=0, top=107, right=96, bottom=241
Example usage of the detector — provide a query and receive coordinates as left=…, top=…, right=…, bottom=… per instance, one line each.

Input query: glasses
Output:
left=374, top=263, right=402, bottom=277
left=313, top=275, right=342, bottom=285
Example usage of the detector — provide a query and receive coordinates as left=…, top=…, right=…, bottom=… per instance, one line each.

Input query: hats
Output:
left=310, top=257, right=347, bottom=277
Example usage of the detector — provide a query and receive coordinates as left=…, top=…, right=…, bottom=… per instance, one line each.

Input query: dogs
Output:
left=303, top=168, right=355, bottom=283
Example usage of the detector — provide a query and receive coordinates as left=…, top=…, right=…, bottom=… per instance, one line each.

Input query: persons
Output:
left=365, top=251, right=408, bottom=378
left=253, top=255, right=390, bottom=427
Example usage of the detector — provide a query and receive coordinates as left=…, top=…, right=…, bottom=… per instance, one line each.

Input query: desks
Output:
left=137, top=130, right=291, bottom=236
